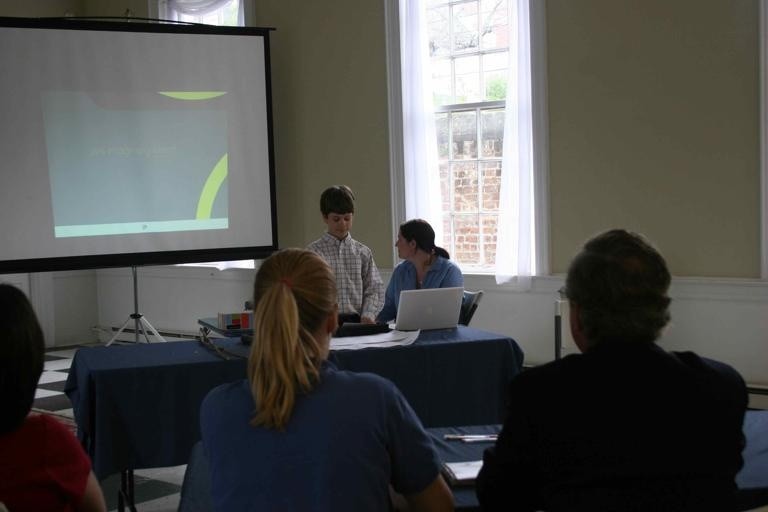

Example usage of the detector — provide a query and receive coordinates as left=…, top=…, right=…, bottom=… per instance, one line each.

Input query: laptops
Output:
left=395, top=287, right=464, bottom=331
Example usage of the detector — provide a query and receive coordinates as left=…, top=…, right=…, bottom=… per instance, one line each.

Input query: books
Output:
left=441, top=460, right=483, bottom=488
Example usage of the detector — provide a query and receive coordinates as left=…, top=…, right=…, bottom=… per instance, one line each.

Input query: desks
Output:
left=72, top=317, right=525, bottom=511
left=423, top=409, right=766, bottom=512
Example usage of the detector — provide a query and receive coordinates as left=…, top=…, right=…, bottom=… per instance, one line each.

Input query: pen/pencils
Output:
left=444, top=434, right=498, bottom=442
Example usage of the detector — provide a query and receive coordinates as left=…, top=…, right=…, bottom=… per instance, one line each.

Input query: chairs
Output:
left=459, top=289, right=483, bottom=326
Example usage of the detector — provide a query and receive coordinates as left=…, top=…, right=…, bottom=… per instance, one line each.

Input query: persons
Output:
left=200, top=249, right=455, bottom=512
left=0, top=283, right=107, bottom=512
left=373, top=219, right=462, bottom=332
left=300, top=184, right=386, bottom=334
left=477, top=228, right=749, bottom=512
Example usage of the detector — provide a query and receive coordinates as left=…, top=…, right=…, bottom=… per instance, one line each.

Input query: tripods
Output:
left=105, top=267, right=167, bottom=347
